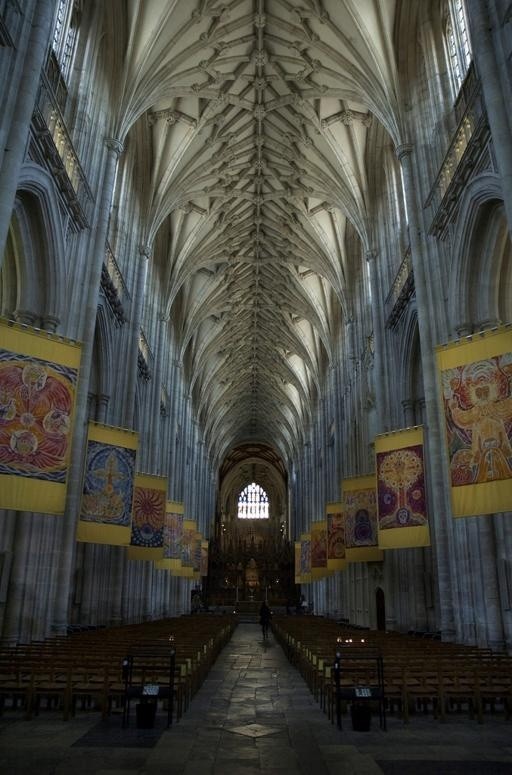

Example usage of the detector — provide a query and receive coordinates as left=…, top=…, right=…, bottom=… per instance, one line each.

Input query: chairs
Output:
left=0, top=614, right=237, bottom=727
left=270, top=612, right=512, bottom=733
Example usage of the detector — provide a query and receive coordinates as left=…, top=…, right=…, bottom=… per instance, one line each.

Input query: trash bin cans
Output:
left=136, top=704, right=157, bottom=729
left=350, top=706, right=372, bottom=732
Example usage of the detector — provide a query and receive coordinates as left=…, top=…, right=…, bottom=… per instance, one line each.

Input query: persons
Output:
left=259, top=600, right=273, bottom=640
